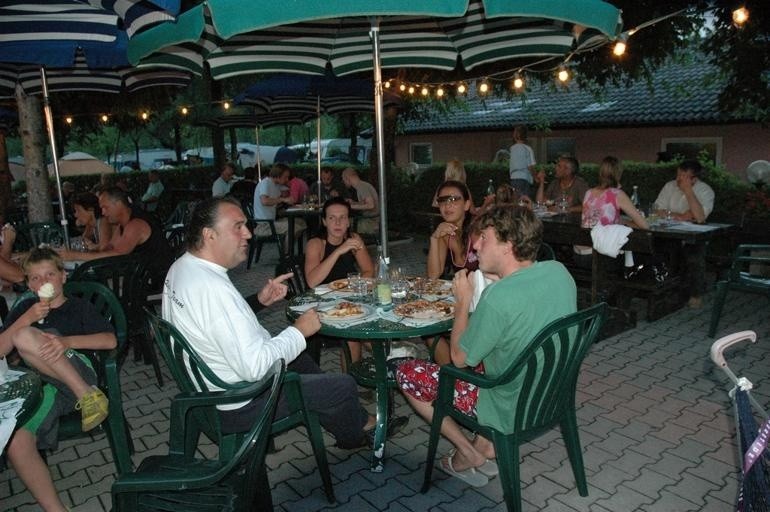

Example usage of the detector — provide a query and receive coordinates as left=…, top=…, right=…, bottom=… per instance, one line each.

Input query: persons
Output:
left=569, top=155, right=650, bottom=309
left=396, top=204, right=578, bottom=471
left=421, top=178, right=487, bottom=364
left=508, top=124, right=540, bottom=202
left=650, top=159, right=717, bottom=310
left=0, top=149, right=409, bottom=512
left=432, top=162, right=496, bottom=217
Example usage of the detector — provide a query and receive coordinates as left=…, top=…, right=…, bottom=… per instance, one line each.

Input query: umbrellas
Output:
left=193, top=102, right=324, bottom=184
left=0, top=1, right=184, bottom=249
left=232, top=70, right=402, bottom=206
left=126, top=0, right=623, bottom=275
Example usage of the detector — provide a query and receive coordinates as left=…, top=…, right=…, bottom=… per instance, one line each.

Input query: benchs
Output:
left=542, top=216, right=674, bottom=320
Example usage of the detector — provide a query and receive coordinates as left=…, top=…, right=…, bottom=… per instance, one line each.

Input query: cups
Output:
left=346, top=272, right=361, bottom=288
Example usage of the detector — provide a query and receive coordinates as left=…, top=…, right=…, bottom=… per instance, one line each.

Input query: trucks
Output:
left=100, top=134, right=373, bottom=172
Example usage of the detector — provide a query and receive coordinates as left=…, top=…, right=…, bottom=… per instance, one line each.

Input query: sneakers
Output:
left=367, top=416, right=409, bottom=445
left=75, top=385, right=109, bottom=433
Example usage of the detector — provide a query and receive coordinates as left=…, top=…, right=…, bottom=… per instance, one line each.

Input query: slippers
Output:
left=440, top=447, right=498, bottom=488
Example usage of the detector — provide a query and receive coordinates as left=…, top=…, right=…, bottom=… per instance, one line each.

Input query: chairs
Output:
left=707, top=244, right=770, bottom=337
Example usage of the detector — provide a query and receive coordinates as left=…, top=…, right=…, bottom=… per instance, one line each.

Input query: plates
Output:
left=316, top=279, right=454, bottom=322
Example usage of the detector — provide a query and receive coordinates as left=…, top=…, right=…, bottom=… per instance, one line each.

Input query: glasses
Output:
left=436, top=195, right=464, bottom=204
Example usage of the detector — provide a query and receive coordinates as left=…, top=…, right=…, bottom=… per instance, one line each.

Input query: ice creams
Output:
left=37, top=281, right=55, bottom=325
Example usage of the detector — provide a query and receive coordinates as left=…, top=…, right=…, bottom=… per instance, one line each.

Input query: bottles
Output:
left=486, top=178, right=494, bottom=195
left=628, top=186, right=642, bottom=209
left=372, top=246, right=392, bottom=310
